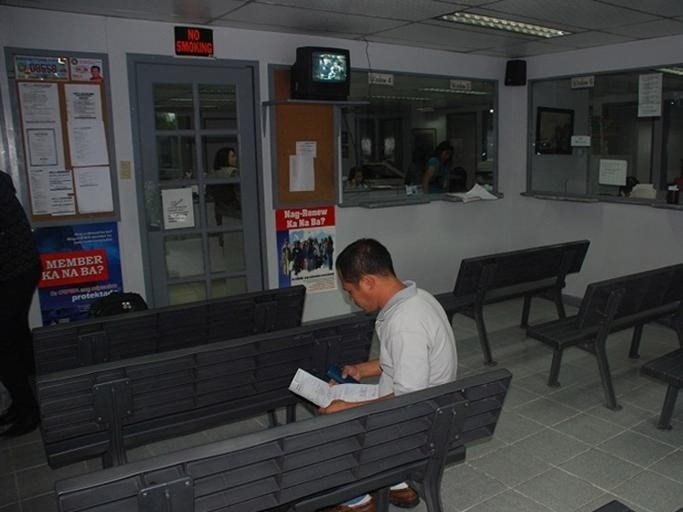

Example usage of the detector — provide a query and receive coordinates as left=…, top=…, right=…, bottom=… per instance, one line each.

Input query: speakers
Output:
left=505, top=60, right=526, bottom=85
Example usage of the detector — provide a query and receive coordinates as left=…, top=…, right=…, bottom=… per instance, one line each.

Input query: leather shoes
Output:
left=331, top=487, right=419, bottom=512
left=0, top=409, right=38, bottom=437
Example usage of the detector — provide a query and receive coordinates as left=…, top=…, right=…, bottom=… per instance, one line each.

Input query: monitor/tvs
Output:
left=291, top=46, right=350, bottom=101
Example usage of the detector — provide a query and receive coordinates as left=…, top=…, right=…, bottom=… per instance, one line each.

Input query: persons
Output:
left=318, top=55, right=346, bottom=80
left=280, top=234, right=332, bottom=277
left=342, top=166, right=368, bottom=190
left=203, top=146, right=240, bottom=249
left=0, top=170, right=43, bottom=442
left=441, top=165, right=466, bottom=192
left=422, top=138, right=452, bottom=194
left=315, top=238, right=459, bottom=512
left=88, top=64, right=103, bottom=81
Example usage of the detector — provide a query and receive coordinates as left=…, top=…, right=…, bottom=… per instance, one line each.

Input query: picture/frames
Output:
left=411, top=127, right=437, bottom=152
